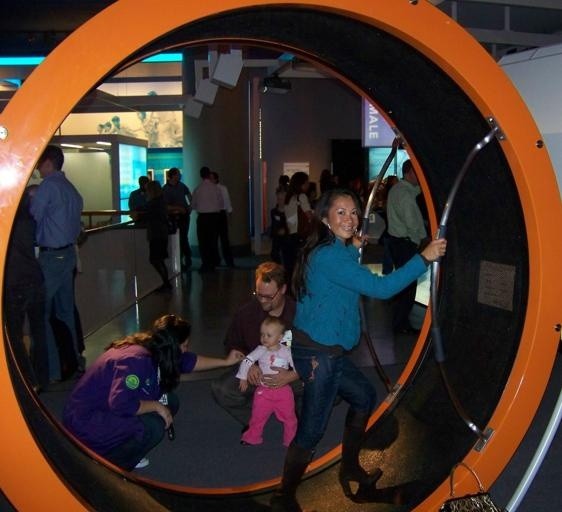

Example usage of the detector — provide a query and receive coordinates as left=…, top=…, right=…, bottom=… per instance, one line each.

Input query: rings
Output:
left=272, top=383, right=276, bottom=386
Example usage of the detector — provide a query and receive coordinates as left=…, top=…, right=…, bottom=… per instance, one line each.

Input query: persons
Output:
left=386, top=159, right=426, bottom=329
left=128, top=167, right=232, bottom=292
left=26, top=183, right=86, bottom=381
left=268, top=188, right=449, bottom=510
left=65, top=314, right=246, bottom=472
left=4, top=201, right=46, bottom=397
left=27, top=144, right=88, bottom=385
left=272, top=168, right=399, bottom=265
left=210, top=261, right=343, bottom=448
left=234, top=316, right=303, bottom=447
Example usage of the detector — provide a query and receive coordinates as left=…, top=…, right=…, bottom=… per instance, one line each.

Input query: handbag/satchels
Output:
left=297, top=192, right=315, bottom=233
left=438, top=462, right=507, bottom=512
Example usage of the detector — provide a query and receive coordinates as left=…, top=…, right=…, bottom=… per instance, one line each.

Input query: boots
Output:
left=338, top=422, right=383, bottom=497
left=269, top=445, right=316, bottom=511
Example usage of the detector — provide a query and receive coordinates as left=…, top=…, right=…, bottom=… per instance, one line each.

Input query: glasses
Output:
left=252, top=288, right=280, bottom=301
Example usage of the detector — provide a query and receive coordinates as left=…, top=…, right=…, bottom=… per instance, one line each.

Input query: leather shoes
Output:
left=398, top=326, right=418, bottom=336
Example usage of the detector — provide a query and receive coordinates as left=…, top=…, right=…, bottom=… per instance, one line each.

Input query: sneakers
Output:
left=133, top=456, right=153, bottom=472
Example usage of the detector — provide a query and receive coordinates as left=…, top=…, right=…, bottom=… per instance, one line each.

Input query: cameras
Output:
left=168, top=426, right=176, bottom=440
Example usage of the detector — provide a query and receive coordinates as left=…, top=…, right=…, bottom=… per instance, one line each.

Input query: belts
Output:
left=40, top=243, right=73, bottom=251
